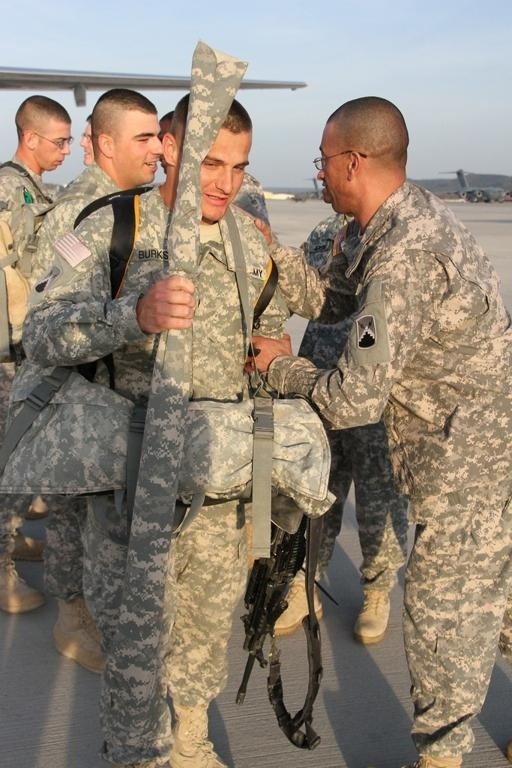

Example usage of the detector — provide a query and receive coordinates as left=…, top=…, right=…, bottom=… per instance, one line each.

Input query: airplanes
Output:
left=0, top=67, right=307, bottom=106
left=439, top=169, right=505, bottom=203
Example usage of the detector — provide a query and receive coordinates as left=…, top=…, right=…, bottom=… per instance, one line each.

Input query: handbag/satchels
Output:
left=0, top=362, right=337, bottom=521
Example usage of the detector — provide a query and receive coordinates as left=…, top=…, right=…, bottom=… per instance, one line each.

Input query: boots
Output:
left=354, top=590, right=390, bottom=644
left=0, top=495, right=47, bottom=613
left=54, top=599, right=108, bottom=674
left=274, top=575, right=322, bottom=635
left=168, top=703, right=227, bottom=768
left=401, top=755, right=463, bottom=768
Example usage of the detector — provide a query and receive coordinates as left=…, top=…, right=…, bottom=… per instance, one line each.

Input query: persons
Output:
left=27, top=87, right=162, bottom=674
left=157, top=111, right=270, bottom=225
left=22, top=93, right=291, bottom=768
left=0, top=95, right=72, bottom=614
left=273, top=212, right=409, bottom=644
left=244, top=96, right=512, bottom=768
left=26, top=115, right=94, bottom=520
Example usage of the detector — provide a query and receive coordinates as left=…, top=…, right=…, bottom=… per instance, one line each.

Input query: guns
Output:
left=235, top=513, right=307, bottom=707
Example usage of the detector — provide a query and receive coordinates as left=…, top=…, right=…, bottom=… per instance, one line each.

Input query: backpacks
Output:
left=0, top=193, right=94, bottom=365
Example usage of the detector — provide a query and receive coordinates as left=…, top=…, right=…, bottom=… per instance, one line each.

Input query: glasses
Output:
left=35, top=133, right=73, bottom=146
left=314, top=150, right=366, bottom=169
